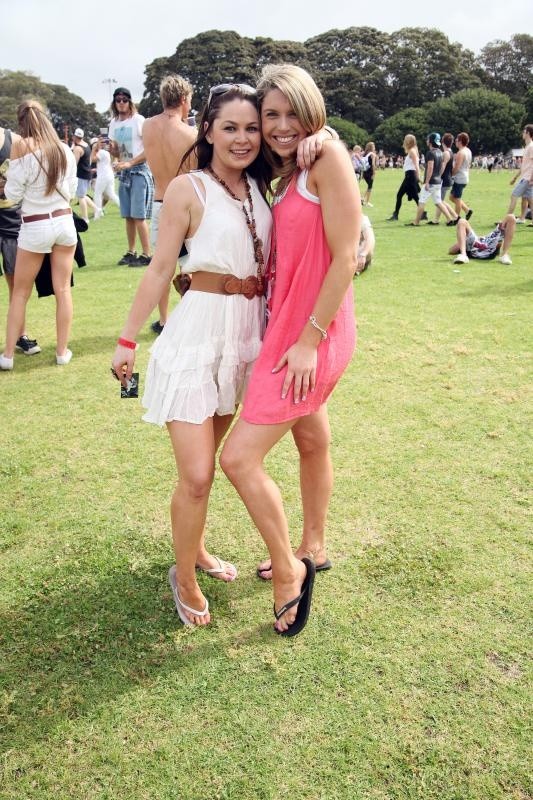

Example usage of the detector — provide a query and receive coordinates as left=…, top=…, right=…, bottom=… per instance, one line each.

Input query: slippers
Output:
left=257, top=559, right=332, bottom=580
left=169, top=565, right=209, bottom=625
left=274, top=558, right=315, bottom=637
left=204, top=555, right=237, bottom=580
left=404, top=223, right=419, bottom=227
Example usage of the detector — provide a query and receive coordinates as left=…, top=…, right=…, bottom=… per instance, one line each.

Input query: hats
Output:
left=113, top=87, right=131, bottom=98
left=430, top=133, right=442, bottom=145
left=71, top=128, right=84, bottom=138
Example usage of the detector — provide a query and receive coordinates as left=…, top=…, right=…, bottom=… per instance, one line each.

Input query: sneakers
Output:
left=0, top=353, right=13, bottom=370
left=129, top=253, right=152, bottom=267
left=119, top=250, right=138, bottom=264
left=499, top=255, right=511, bottom=264
left=17, top=336, right=41, bottom=354
left=56, top=349, right=72, bottom=365
left=454, top=255, right=468, bottom=264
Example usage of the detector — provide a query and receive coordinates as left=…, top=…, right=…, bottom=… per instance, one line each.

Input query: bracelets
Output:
left=309, top=315, right=327, bottom=341
left=118, top=337, right=136, bottom=349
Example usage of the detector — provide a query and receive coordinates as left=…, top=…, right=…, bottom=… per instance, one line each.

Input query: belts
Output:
left=23, top=208, right=71, bottom=222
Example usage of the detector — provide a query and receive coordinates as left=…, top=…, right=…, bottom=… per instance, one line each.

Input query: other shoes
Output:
left=365, top=203, right=372, bottom=207
left=386, top=215, right=398, bottom=221
left=516, top=218, right=524, bottom=223
left=421, top=211, right=473, bottom=227
left=151, top=320, right=164, bottom=335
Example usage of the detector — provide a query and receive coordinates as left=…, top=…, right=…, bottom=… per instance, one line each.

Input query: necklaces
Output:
left=207, top=163, right=263, bottom=285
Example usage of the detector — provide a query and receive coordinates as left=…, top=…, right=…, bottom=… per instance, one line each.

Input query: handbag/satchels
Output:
left=361, top=153, right=372, bottom=171
left=352, top=153, right=360, bottom=171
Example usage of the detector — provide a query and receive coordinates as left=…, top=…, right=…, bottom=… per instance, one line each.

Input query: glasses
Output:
left=116, top=97, right=127, bottom=103
left=207, top=84, right=256, bottom=108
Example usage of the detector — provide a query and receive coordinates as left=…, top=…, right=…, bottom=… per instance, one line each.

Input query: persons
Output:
left=0, top=76, right=533, bottom=370
left=220, top=63, right=363, bottom=639
left=110, top=83, right=334, bottom=628
